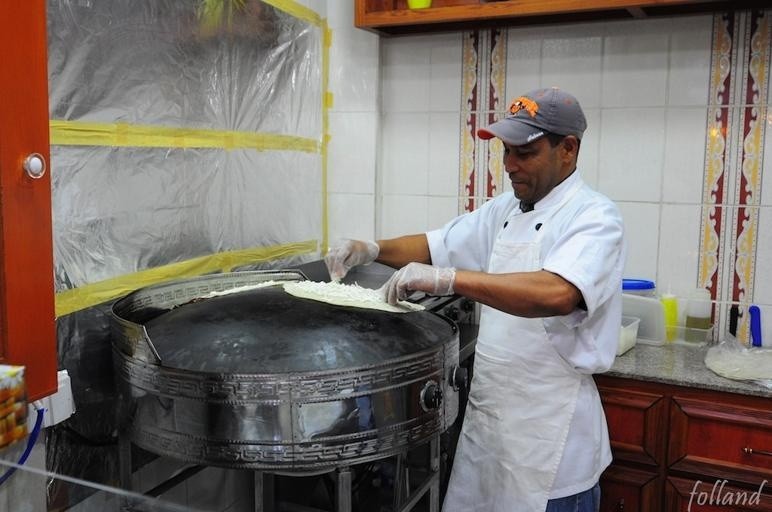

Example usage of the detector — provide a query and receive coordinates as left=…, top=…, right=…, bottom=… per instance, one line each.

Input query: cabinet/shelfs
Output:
left=662, top=278, right=678, bottom=341
left=687, top=286, right=711, bottom=343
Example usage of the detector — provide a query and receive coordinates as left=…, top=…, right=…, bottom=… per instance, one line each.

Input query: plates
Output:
left=597, top=378, right=769, bottom=512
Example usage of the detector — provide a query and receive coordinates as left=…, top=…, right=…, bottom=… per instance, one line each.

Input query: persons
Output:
left=325, top=85, right=623, bottom=511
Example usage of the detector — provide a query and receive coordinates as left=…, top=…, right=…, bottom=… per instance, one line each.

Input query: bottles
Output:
left=477, top=87, right=587, bottom=146
